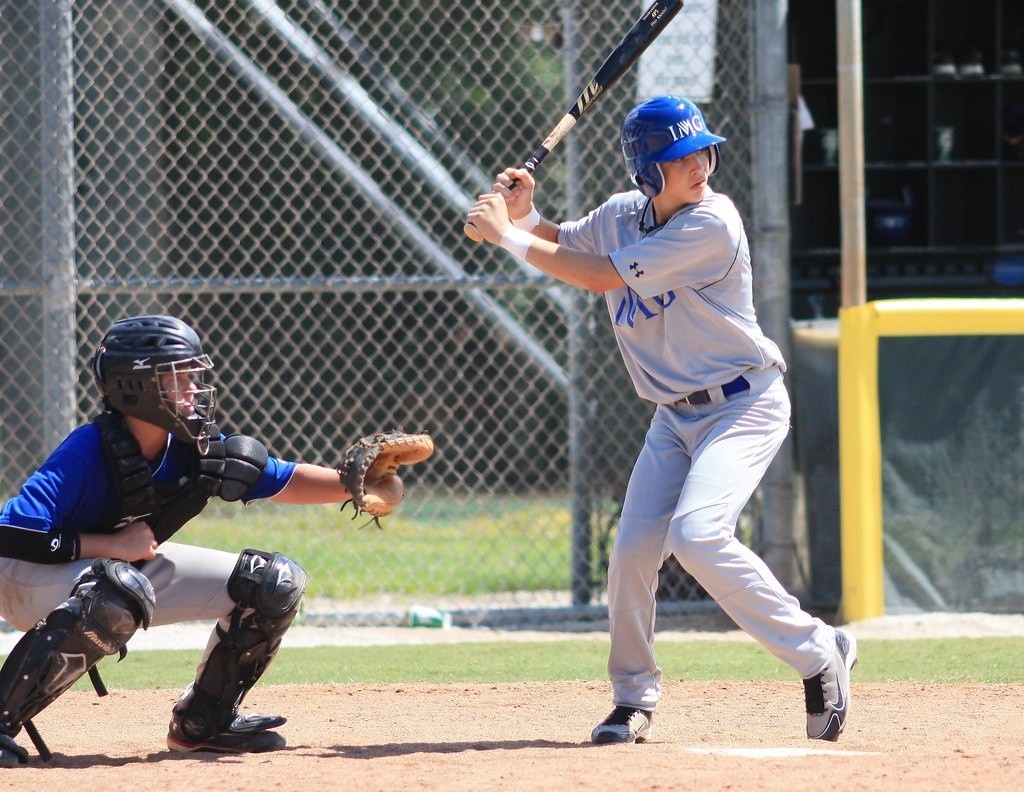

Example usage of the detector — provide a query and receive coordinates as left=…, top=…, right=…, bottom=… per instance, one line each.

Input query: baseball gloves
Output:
left=339, top=432, right=436, bottom=518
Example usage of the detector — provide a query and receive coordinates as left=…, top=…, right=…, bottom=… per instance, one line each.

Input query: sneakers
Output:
left=0, top=735, right=29, bottom=766
left=803, top=630, right=859, bottom=740
left=591, top=705, right=653, bottom=745
left=167, top=707, right=288, bottom=753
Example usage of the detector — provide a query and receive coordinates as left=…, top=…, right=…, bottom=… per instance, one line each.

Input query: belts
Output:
left=676, top=376, right=751, bottom=405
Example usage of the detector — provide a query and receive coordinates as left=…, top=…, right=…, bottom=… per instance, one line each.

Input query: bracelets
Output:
left=499, top=223, right=536, bottom=260
left=508, top=204, right=540, bottom=231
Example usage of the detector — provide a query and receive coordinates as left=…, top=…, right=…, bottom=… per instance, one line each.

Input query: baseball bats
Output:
left=464, top=0, right=685, bottom=244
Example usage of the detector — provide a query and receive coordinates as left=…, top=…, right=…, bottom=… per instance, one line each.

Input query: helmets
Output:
left=95, top=315, right=219, bottom=455
left=621, top=95, right=726, bottom=197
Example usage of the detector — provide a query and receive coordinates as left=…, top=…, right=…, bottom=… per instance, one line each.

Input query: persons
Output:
left=465, top=95, right=860, bottom=746
left=1, top=315, right=434, bottom=770
